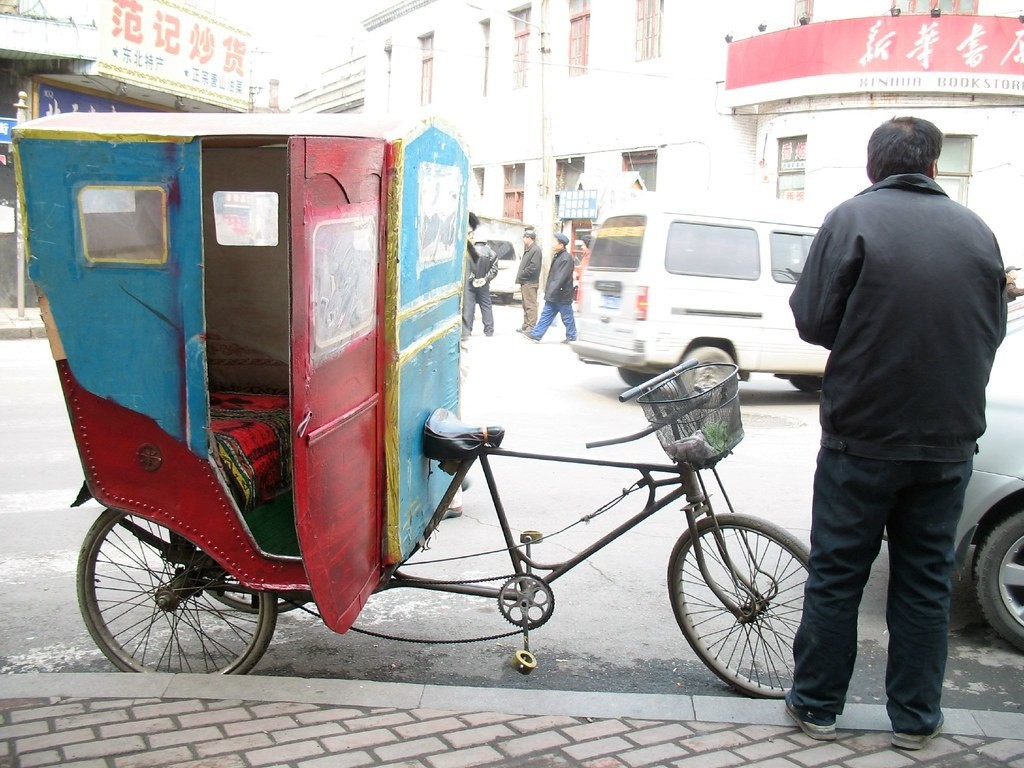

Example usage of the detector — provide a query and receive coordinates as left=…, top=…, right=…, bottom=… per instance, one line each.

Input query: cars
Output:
left=880, top=296, right=1024, bottom=656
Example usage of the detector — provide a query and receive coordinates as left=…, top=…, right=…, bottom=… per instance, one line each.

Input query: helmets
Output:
left=473, top=228, right=488, bottom=244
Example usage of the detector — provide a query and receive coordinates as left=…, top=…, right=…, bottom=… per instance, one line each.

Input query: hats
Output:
left=582, top=235, right=592, bottom=248
left=522, top=230, right=536, bottom=240
left=1006, top=266, right=1022, bottom=274
left=554, top=233, right=569, bottom=246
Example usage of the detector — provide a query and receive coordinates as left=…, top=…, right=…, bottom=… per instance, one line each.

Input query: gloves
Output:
left=472, top=277, right=485, bottom=288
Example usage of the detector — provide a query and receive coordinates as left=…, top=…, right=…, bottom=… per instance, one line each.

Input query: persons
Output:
left=515, top=231, right=542, bottom=332
left=460, top=212, right=479, bottom=340
left=573, top=234, right=595, bottom=304
left=522, top=233, right=577, bottom=344
left=786, top=115, right=1007, bottom=750
left=469, top=228, right=499, bottom=337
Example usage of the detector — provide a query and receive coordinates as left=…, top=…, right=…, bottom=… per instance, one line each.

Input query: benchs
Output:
left=207, top=390, right=290, bottom=508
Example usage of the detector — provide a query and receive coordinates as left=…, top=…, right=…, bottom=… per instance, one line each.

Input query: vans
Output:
left=564, top=199, right=832, bottom=393
left=477, top=232, right=523, bottom=304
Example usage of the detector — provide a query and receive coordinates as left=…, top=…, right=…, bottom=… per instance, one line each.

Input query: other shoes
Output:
left=892, top=711, right=945, bottom=749
left=561, top=339, right=574, bottom=344
left=523, top=332, right=539, bottom=344
left=785, top=689, right=836, bottom=739
left=516, top=329, right=528, bottom=333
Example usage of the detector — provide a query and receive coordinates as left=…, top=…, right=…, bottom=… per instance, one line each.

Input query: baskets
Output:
left=636, top=363, right=744, bottom=468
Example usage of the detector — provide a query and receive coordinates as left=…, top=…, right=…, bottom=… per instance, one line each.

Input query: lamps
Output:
left=798, top=12, right=813, bottom=26
left=757, top=20, right=769, bottom=33
left=724, top=30, right=736, bottom=44
left=882, top=4, right=902, bottom=18
left=994, top=9, right=1024, bottom=23
left=931, top=3, right=942, bottom=17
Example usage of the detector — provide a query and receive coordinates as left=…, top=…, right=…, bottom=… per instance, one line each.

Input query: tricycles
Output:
left=8, top=108, right=810, bottom=699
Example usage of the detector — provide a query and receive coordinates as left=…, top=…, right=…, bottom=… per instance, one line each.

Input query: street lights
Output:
left=466, top=1, right=549, bottom=293
